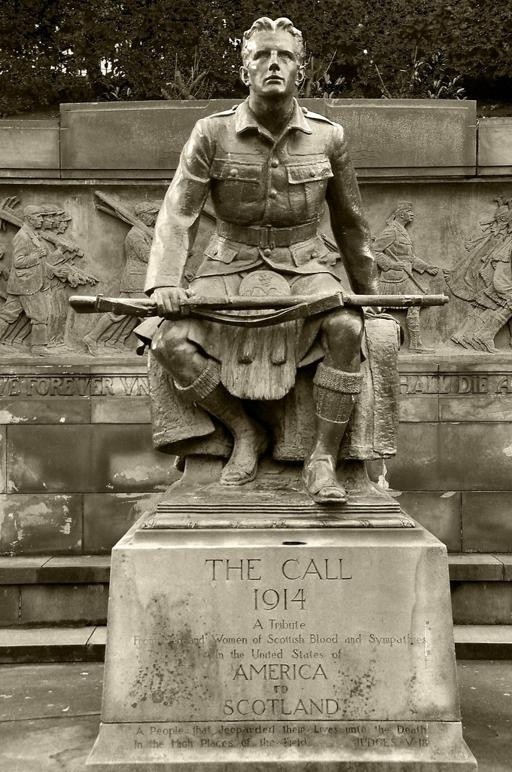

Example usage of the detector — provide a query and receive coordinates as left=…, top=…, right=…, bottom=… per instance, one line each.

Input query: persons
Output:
left=374, top=196, right=512, bottom=355
left=143, top=16, right=384, bottom=505
left=0, top=192, right=164, bottom=355
